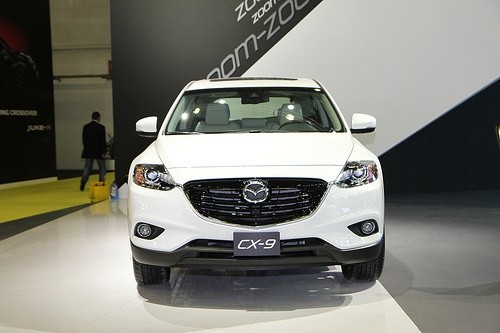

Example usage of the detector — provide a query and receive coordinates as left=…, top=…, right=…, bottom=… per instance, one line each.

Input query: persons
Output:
left=79, top=111, right=107, bottom=191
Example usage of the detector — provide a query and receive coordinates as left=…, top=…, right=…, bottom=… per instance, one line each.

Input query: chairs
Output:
left=241, top=118, right=267, bottom=129
left=271, top=103, right=316, bottom=130
left=194, top=103, right=242, bottom=131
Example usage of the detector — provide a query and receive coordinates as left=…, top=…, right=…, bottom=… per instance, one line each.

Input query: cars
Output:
left=127, top=77, right=386, bottom=286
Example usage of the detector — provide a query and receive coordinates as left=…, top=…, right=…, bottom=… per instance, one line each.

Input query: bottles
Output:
left=110, top=183, right=118, bottom=199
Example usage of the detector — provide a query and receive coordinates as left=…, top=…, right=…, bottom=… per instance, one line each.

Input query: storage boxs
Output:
left=90, top=182, right=109, bottom=199
